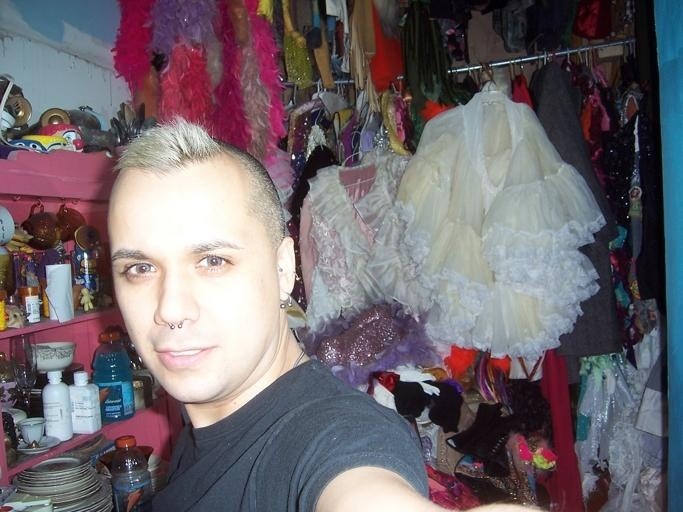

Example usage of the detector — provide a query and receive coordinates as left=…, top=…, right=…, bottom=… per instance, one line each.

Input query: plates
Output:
left=98, top=444, right=169, bottom=495
left=11, top=452, right=111, bottom=511
left=17, top=435, right=61, bottom=455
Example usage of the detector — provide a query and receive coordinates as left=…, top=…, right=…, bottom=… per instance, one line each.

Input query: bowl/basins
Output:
left=0, top=204, right=15, bottom=246
left=23, top=339, right=76, bottom=374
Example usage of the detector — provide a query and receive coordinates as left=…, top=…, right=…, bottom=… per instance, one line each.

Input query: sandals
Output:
left=446, top=403, right=503, bottom=454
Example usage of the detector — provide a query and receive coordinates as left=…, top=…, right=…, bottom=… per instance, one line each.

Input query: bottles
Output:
left=88, top=330, right=134, bottom=426
left=0, top=353, right=12, bottom=385
left=40, top=371, right=73, bottom=442
left=111, top=434, right=156, bottom=512
left=67, top=371, right=101, bottom=435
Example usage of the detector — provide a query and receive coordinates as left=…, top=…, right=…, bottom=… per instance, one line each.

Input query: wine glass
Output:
left=11, top=361, right=37, bottom=413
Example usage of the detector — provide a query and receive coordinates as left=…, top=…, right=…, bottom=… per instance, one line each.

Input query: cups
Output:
left=20, top=203, right=86, bottom=249
left=36, top=124, right=83, bottom=153
left=0, top=294, right=8, bottom=331
left=15, top=285, right=40, bottom=324
left=16, top=416, right=46, bottom=446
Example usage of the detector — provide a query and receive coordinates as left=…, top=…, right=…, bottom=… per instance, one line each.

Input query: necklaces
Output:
left=293, top=350, right=305, bottom=368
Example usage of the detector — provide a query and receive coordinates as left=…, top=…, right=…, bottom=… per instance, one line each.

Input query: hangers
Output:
left=269, top=40, right=646, bottom=110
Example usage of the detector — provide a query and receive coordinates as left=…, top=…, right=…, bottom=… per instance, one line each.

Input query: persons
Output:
left=106, top=114, right=542, bottom=512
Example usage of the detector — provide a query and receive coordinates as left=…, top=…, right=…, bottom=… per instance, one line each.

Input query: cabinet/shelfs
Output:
left=0, top=148, right=187, bottom=512
